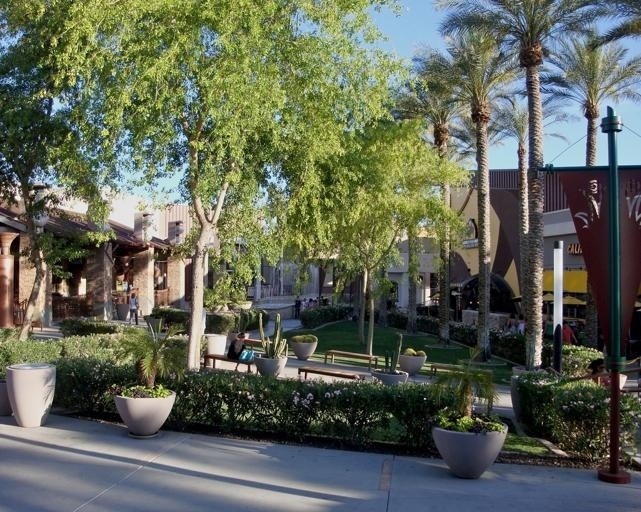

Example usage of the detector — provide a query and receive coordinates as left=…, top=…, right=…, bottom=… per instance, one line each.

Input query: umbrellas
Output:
left=543, top=293, right=586, bottom=317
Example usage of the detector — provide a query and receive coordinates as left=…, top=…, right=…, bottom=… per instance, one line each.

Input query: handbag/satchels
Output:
left=240, top=351, right=255, bottom=363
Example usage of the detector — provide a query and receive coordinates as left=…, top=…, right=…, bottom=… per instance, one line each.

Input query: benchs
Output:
left=203, top=353, right=255, bottom=372
left=298, top=366, right=362, bottom=383
left=325, top=349, right=378, bottom=371
left=243, top=339, right=289, bottom=355
left=432, top=363, right=493, bottom=387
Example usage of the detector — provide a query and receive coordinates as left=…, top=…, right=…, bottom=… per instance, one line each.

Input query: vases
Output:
left=6, top=364, right=57, bottom=428
left=0, top=382, right=13, bottom=416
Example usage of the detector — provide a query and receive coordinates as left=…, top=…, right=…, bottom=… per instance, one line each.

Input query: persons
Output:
left=294, top=296, right=301, bottom=320
left=128, top=293, right=139, bottom=325
left=506, top=313, right=528, bottom=336
left=563, top=321, right=578, bottom=345
left=303, top=297, right=319, bottom=311
left=591, top=358, right=609, bottom=381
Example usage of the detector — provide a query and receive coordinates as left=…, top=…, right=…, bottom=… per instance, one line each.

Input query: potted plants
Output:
left=255, top=313, right=288, bottom=379
left=398, top=348, right=427, bottom=375
left=373, top=352, right=408, bottom=385
left=111, top=316, right=185, bottom=439
left=511, top=376, right=565, bottom=437
left=430, top=346, right=510, bottom=478
left=204, top=314, right=236, bottom=355
left=290, top=334, right=317, bottom=361
